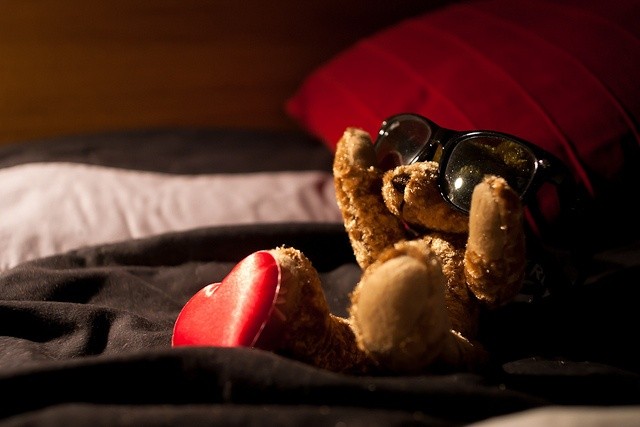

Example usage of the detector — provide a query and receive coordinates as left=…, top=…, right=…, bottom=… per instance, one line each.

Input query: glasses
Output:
left=369, top=113, right=584, bottom=217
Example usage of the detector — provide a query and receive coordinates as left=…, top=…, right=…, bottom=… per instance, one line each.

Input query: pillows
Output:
left=287, top=0, right=640, bottom=248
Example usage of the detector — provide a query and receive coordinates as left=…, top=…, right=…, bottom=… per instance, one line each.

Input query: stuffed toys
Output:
left=253, top=112, right=565, bottom=374
